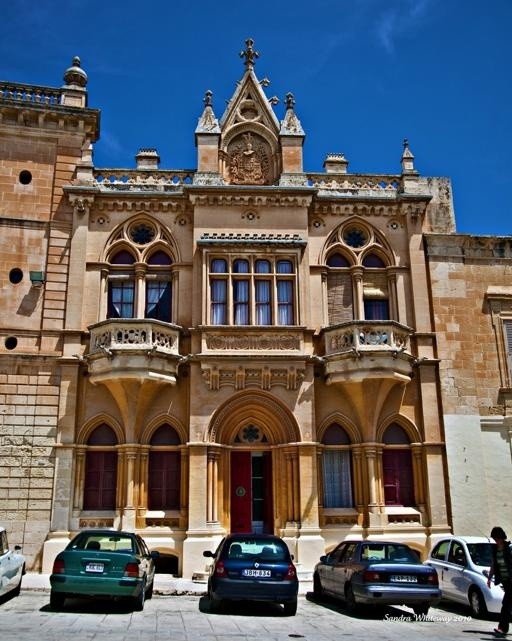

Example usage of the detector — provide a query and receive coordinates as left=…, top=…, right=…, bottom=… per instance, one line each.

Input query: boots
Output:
left=494, top=626, right=506, bottom=635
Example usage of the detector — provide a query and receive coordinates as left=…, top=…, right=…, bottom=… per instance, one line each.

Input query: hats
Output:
left=491, top=527, right=507, bottom=540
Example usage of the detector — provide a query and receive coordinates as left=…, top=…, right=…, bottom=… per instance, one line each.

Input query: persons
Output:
left=488, top=524, right=512, bottom=637
